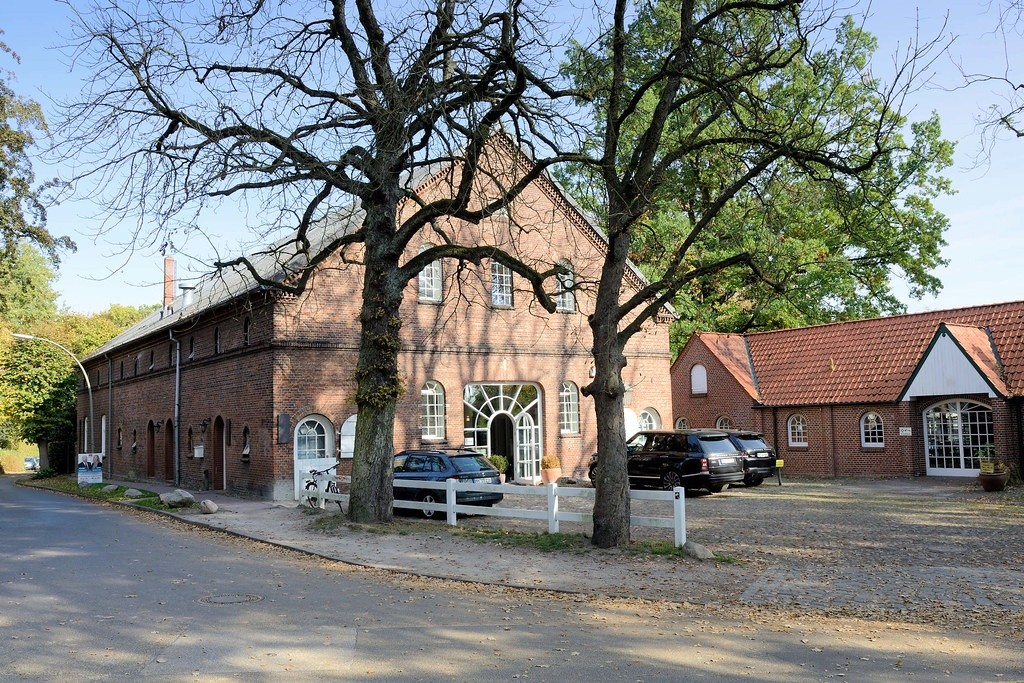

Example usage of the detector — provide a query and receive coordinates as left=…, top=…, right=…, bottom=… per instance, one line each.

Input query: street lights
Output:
left=11, top=333, right=94, bottom=452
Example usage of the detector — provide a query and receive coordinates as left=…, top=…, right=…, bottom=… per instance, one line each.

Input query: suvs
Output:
left=393, top=448, right=503, bottom=519
left=588, top=428, right=777, bottom=492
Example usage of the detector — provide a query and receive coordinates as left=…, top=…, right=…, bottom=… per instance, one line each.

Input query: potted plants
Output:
left=541, top=454, right=561, bottom=485
left=490, top=454, right=509, bottom=484
left=974, top=443, right=1010, bottom=492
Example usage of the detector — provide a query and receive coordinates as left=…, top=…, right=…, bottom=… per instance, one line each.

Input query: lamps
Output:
left=153, top=420, right=163, bottom=433
left=199, top=418, right=210, bottom=434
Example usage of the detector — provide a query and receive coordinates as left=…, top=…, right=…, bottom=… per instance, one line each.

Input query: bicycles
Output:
left=305, top=461, right=343, bottom=514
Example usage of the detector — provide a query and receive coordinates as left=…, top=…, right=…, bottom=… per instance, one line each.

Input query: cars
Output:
left=24, top=457, right=40, bottom=470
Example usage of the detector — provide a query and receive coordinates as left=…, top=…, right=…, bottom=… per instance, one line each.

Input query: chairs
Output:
left=653, top=437, right=663, bottom=449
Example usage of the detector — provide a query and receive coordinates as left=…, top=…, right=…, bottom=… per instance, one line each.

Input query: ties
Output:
left=85, top=462, right=87, bottom=468
